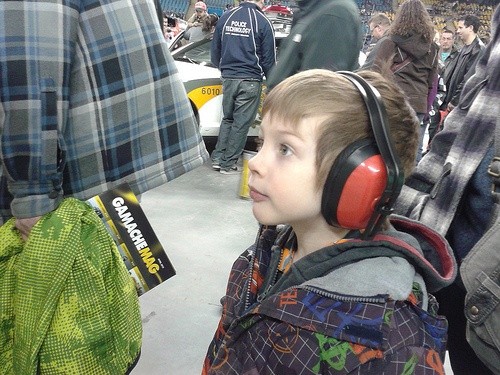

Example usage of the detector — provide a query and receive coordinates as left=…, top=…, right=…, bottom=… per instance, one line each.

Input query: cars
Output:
left=168, top=5, right=294, bottom=155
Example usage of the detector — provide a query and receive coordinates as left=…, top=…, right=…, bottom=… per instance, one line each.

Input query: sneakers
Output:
left=212, top=160, right=221, bottom=169
left=219, top=166, right=242, bottom=175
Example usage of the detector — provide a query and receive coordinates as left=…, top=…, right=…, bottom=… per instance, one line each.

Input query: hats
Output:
left=195, top=1, right=207, bottom=10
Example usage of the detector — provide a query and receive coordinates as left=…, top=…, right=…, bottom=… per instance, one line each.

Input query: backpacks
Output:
left=0, top=196, right=143, bottom=375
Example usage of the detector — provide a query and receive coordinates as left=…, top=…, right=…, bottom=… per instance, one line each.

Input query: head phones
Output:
left=322, top=69, right=404, bottom=240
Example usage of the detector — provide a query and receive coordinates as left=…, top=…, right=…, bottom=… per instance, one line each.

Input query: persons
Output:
left=160, top=0, right=485, bottom=172
left=391, top=1, right=500, bottom=375
left=0, top=0, right=210, bottom=243
left=202, top=69, right=458, bottom=375
